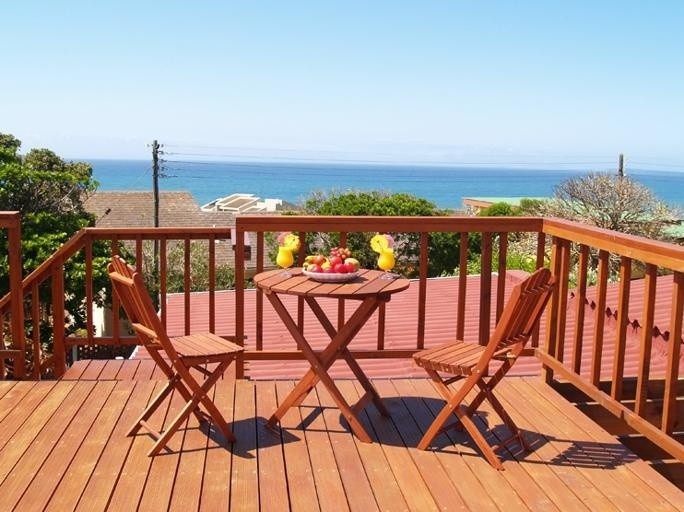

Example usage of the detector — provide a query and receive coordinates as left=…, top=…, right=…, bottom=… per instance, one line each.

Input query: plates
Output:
left=301, top=267, right=362, bottom=282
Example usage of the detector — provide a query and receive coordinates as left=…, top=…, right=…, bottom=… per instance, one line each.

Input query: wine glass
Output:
left=276, top=246, right=294, bottom=279
left=376, top=248, right=395, bottom=280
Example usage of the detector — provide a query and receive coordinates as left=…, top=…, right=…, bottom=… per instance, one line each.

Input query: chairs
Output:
left=412, top=267, right=556, bottom=472
left=106, top=255, right=244, bottom=457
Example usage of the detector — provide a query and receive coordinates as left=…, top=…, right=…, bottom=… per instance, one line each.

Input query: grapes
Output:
left=329, top=247, right=352, bottom=259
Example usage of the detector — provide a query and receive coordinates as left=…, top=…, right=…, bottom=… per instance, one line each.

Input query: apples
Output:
left=307, top=255, right=360, bottom=273
